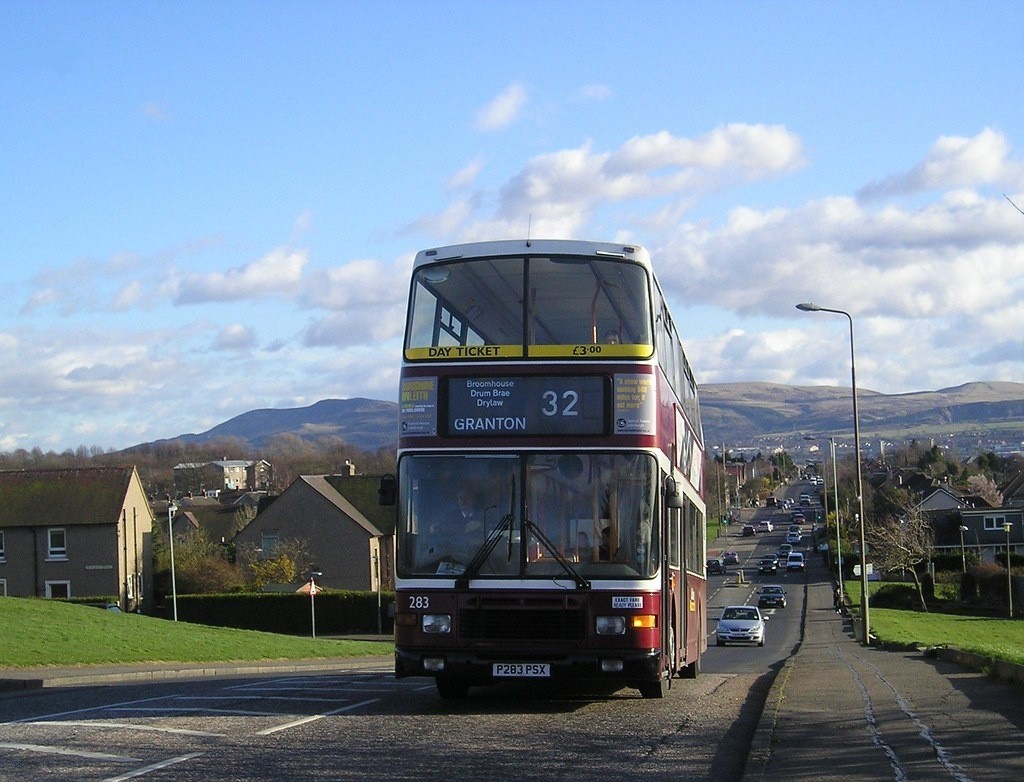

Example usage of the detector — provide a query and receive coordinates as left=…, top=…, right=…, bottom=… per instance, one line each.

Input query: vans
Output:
left=785, top=553, right=806, bottom=570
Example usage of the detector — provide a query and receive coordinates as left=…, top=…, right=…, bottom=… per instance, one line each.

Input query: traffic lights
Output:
left=723, top=515, right=727, bottom=524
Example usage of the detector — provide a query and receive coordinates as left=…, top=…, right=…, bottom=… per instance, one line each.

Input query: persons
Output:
left=832, top=578, right=845, bottom=606
left=604, top=330, right=619, bottom=344
left=578, top=502, right=652, bottom=562
left=429, top=489, right=492, bottom=538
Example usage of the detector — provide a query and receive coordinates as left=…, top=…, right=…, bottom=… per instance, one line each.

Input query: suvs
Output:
left=766, top=497, right=778, bottom=507
left=799, top=495, right=813, bottom=505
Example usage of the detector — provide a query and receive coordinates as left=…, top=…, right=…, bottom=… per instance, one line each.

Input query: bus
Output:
left=376, top=240, right=706, bottom=708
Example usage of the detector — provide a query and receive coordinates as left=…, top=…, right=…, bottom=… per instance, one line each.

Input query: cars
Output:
left=749, top=498, right=760, bottom=507
left=801, top=472, right=824, bottom=485
left=777, top=545, right=795, bottom=557
left=779, top=498, right=795, bottom=509
left=756, top=561, right=778, bottom=574
left=707, top=560, right=727, bottom=574
left=785, top=532, right=802, bottom=544
left=794, top=514, right=806, bottom=525
left=790, top=511, right=802, bottom=521
left=758, top=521, right=774, bottom=533
left=761, top=554, right=779, bottom=563
left=757, top=585, right=788, bottom=608
left=722, top=554, right=738, bottom=565
left=742, top=525, right=756, bottom=536
left=787, top=525, right=803, bottom=535
left=87, top=603, right=124, bottom=612
left=714, top=605, right=769, bottom=648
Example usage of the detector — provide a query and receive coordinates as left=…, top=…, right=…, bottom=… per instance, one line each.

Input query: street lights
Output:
left=310, top=572, right=322, bottom=638
left=806, top=458, right=831, bottom=570
left=794, top=303, right=870, bottom=649
left=714, top=446, right=733, bottom=537
left=169, top=506, right=179, bottom=621
left=960, top=526, right=968, bottom=571
left=804, top=436, right=845, bottom=612
left=1002, top=522, right=1013, bottom=617
